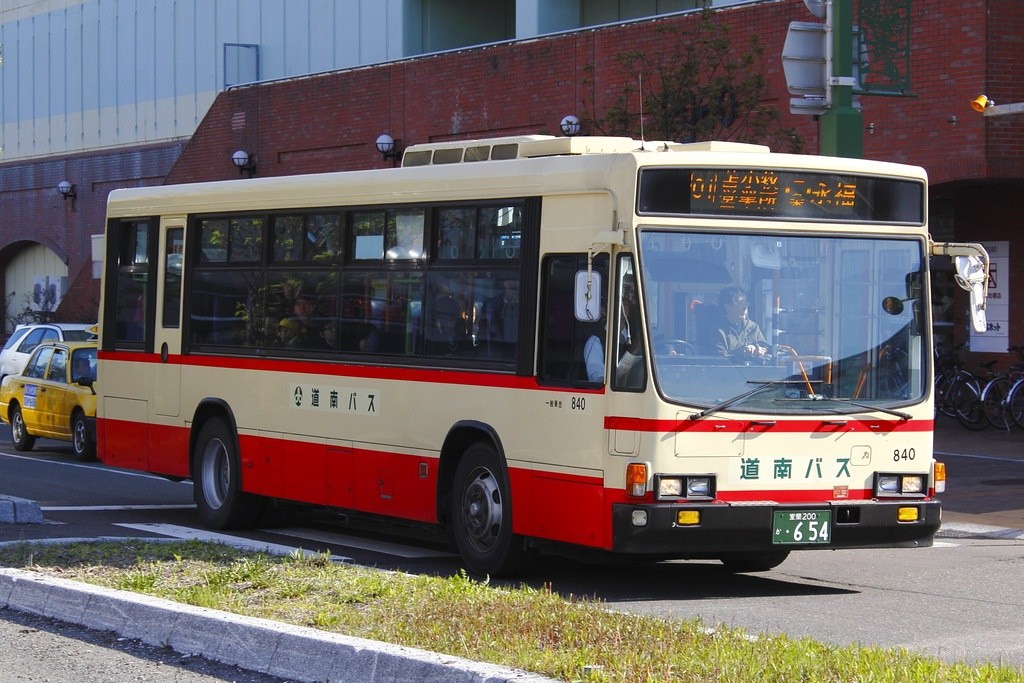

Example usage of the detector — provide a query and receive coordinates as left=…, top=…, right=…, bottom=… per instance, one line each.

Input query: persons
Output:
left=261, top=272, right=519, bottom=359
left=887, top=298, right=899, bottom=314
left=706, top=284, right=772, bottom=364
left=579, top=295, right=676, bottom=387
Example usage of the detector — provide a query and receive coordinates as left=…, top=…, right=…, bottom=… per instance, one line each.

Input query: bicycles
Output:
left=874, top=338, right=1024, bottom=432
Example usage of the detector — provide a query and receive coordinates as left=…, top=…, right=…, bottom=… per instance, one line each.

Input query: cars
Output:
left=0, top=323, right=98, bottom=461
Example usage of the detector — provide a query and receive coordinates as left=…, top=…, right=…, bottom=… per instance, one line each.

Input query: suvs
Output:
left=0, top=323, right=97, bottom=380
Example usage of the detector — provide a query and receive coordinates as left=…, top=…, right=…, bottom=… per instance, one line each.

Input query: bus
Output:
left=93, top=131, right=990, bottom=581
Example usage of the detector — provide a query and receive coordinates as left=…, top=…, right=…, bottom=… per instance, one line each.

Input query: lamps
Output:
left=560, top=116, right=581, bottom=136
left=231, top=151, right=256, bottom=174
left=58, top=180, right=77, bottom=200
left=376, top=134, right=402, bottom=161
